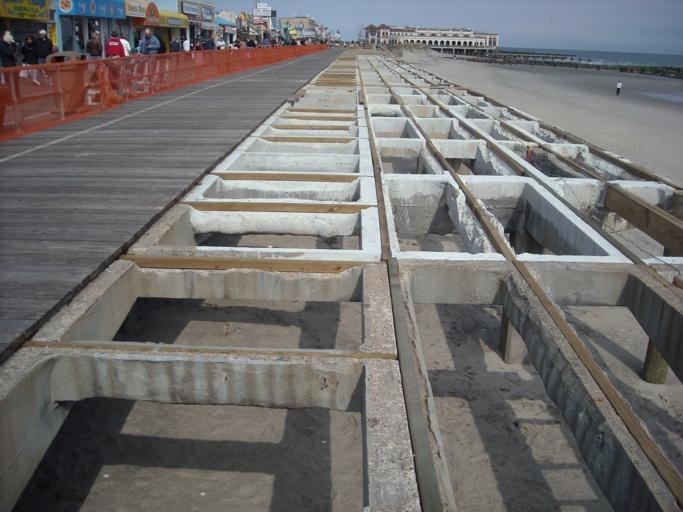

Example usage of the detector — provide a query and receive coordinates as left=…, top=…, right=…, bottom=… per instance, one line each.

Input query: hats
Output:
left=38, top=29, right=46, bottom=34
left=3, top=31, right=15, bottom=43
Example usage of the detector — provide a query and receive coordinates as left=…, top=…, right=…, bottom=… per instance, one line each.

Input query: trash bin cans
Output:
left=45, top=52, right=89, bottom=113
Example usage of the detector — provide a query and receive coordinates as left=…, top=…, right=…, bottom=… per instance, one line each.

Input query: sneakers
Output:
left=31, top=80, right=41, bottom=85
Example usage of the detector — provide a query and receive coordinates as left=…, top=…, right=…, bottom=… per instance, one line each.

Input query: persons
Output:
left=615, top=79, right=622, bottom=96
left=193, top=33, right=349, bottom=72
left=0, top=28, right=193, bottom=131
